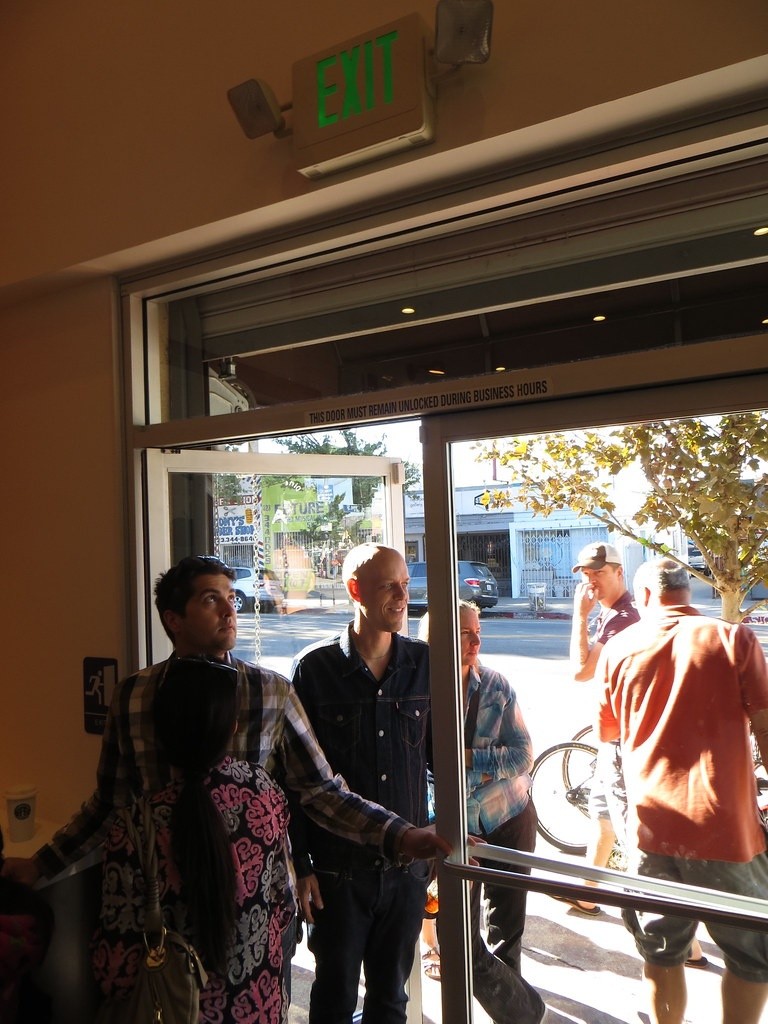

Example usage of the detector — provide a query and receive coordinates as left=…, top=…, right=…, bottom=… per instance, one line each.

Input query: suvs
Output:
left=688, top=547, right=711, bottom=577
left=229, top=566, right=285, bottom=613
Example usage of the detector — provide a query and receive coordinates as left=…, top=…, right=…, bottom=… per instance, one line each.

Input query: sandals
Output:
left=421, top=943, right=441, bottom=978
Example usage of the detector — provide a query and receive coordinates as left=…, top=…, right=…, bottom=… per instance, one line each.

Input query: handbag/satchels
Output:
left=114, top=795, right=209, bottom=1024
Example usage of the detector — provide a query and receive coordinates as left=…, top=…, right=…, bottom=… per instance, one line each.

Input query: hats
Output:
left=572, top=542, right=622, bottom=573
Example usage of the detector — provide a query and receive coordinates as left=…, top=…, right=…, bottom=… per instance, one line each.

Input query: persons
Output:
left=419, top=601, right=538, bottom=974
left=275, top=542, right=436, bottom=1024
left=552, top=542, right=708, bottom=968
left=0, top=557, right=487, bottom=1024
left=596, top=557, right=767, bottom=1024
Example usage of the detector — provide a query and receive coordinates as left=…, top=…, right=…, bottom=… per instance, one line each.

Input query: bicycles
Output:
left=527, top=724, right=768, bottom=855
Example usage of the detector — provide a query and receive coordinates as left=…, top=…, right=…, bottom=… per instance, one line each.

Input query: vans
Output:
left=401, top=561, right=500, bottom=615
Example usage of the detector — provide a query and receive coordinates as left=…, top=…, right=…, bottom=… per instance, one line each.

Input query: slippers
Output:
left=544, top=891, right=599, bottom=916
left=683, top=957, right=709, bottom=968
